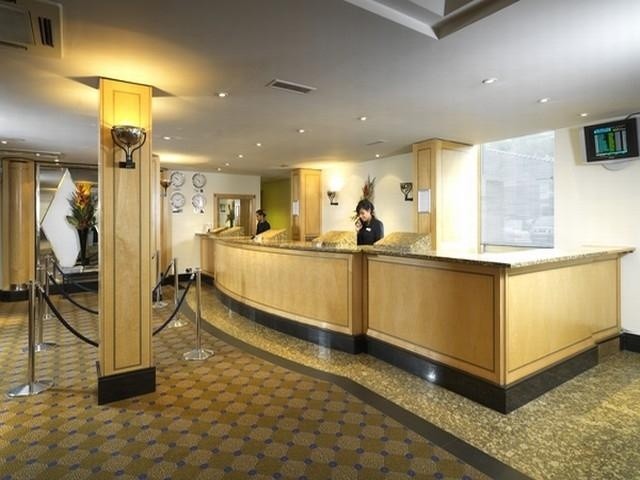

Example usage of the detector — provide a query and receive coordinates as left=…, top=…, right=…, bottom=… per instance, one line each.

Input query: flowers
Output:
left=63, top=182, right=99, bottom=231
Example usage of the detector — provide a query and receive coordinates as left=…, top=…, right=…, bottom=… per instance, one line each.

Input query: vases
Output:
left=73, top=227, right=91, bottom=264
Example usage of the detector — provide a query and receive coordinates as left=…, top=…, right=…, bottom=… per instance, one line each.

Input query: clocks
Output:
left=169, top=169, right=207, bottom=214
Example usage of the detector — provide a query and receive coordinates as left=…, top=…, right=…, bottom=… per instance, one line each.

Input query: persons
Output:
left=357, top=198, right=384, bottom=246
left=254, top=208, right=271, bottom=236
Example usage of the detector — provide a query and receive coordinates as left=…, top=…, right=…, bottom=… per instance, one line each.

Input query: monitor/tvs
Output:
left=578, top=115, right=640, bottom=165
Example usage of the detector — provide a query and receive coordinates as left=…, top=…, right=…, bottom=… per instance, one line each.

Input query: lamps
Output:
left=326, top=189, right=338, bottom=205
left=161, top=179, right=172, bottom=196
left=110, top=124, right=146, bottom=169
left=399, top=182, right=413, bottom=201
